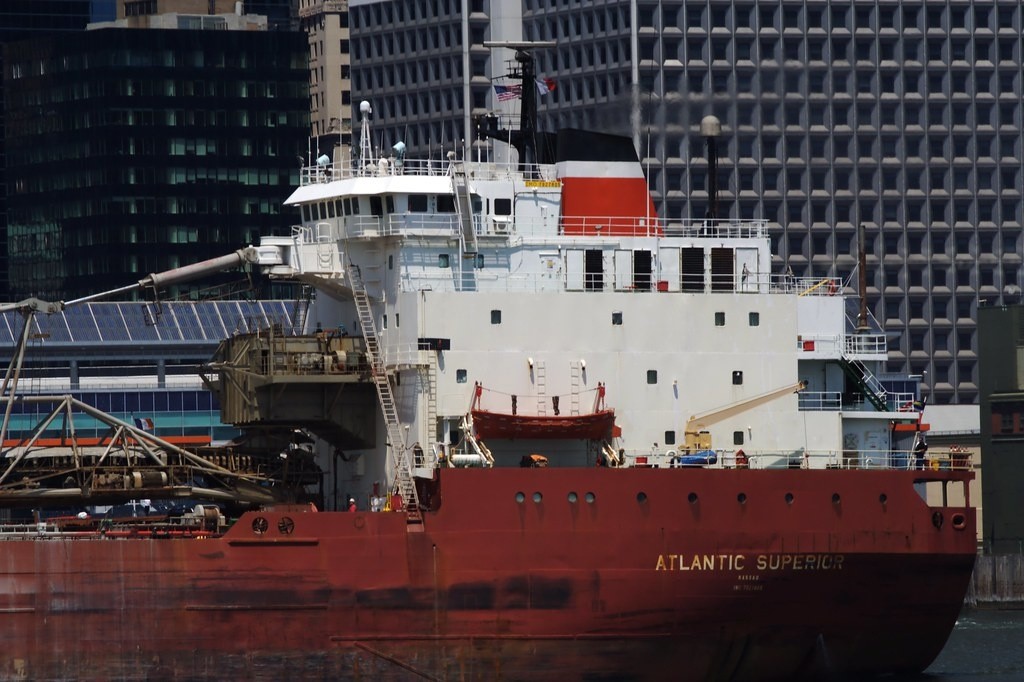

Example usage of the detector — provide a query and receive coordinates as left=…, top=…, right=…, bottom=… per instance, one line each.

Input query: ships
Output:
left=0, top=41, right=977, bottom=682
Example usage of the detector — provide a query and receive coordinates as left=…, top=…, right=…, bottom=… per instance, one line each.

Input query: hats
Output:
left=350, top=498, right=355, bottom=502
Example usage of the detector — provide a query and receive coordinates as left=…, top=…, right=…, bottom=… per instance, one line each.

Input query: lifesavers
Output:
left=827, top=281, right=836, bottom=297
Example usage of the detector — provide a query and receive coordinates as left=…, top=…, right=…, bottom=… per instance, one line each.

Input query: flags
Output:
left=896, top=399, right=925, bottom=424
left=494, top=84, right=522, bottom=102
left=535, top=79, right=556, bottom=95
left=134, top=417, right=154, bottom=430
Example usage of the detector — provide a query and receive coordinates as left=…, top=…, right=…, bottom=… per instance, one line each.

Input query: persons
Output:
left=914, top=437, right=928, bottom=470
left=342, top=494, right=356, bottom=512
left=309, top=501, right=318, bottom=512
left=948, top=444, right=969, bottom=467
left=371, top=496, right=382, bottom=512
